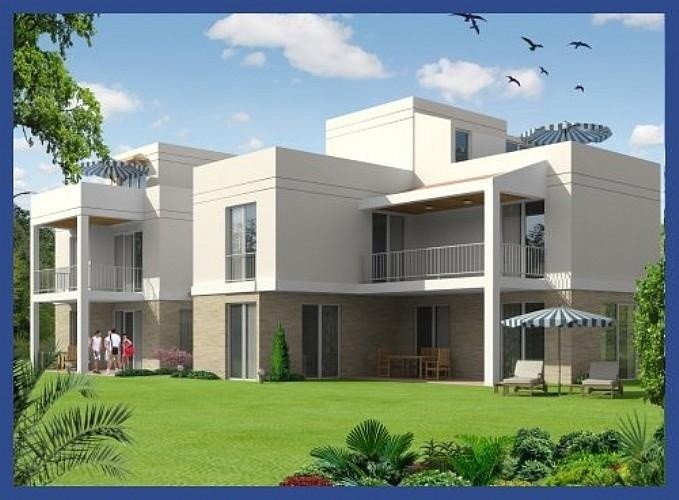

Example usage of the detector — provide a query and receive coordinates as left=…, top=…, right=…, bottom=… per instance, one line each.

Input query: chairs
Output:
left=580, top=361, right=625, bottom=400
left=377, top=347, right=452, bottom=383
left=495, top=359, right=549, bottom=398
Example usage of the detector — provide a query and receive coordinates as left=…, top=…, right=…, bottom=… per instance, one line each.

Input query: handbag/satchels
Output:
left=113, top=347, right=118, bottom=354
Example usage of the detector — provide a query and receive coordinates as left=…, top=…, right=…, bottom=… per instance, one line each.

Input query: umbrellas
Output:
left=502, top=299, right=620, bottom=395
left=518, top=119, right=614, bottom=146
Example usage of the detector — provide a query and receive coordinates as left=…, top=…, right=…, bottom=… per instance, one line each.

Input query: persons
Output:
left=120, top=333, right=133, bottom=371
left=90, top=330, right=103, bottom=373
left=105, top=328, right=121, bottom=375
left=103, top=331, right=119, bottom=372
